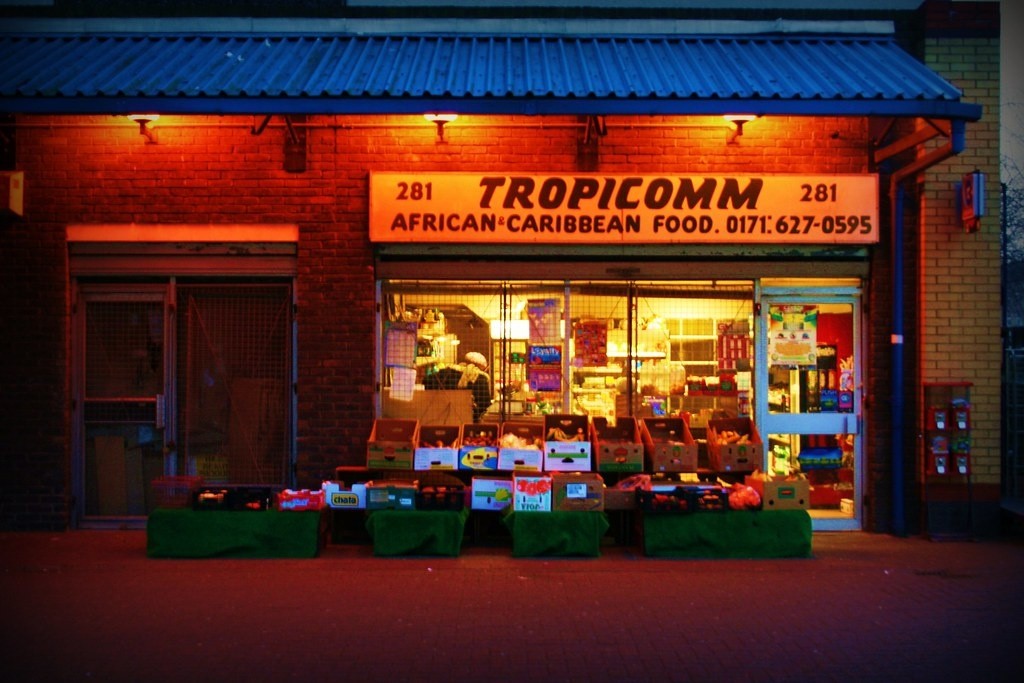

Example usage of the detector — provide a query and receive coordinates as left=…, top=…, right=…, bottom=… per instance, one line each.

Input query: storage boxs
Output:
left=321, top=413, right=644, bottom=513
left=277, top=489, right=325, bottom=511
left=693, top=489, right=729, bottom=512
left=706, top=417, right=764, bottom=472
left=641, top=418, right=698, bottom=472
left=760, top=473, right=810, bottom=510
left=636, top=486, right=692, bottom=513
left=150, top=475, right=274, bottom=511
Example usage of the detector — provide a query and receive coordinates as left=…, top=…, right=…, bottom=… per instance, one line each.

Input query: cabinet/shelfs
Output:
left=492, top=341, right=672, bottom=422
left=389, top=329, right=443, bottom=390
left=767, top=339, right=844, bottom=477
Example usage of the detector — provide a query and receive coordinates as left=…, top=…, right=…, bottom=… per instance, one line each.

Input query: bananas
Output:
left=546, top=428, right=581, bottom=442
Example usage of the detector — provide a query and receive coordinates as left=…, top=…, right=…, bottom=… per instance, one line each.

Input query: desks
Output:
left=147, top=506, right=329, bottom=559
left=503, top=510, right=611, bottom=560
left=366, top=508, right=470, bottom=557
left=637, top=508, right=814, bottom=560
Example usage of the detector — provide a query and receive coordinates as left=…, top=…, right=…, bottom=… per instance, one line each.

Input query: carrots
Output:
left=713, top=427, right=749, bottom=444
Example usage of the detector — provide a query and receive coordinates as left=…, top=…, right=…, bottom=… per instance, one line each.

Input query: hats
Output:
left=465, top=352, right=487, bottom=370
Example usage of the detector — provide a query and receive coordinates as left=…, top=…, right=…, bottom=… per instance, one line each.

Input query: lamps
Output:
left=126, top=115, right=159, bottom=143
left=423, top=113, right=458, bottom=142
left=723, top=115, right=757, bottom=136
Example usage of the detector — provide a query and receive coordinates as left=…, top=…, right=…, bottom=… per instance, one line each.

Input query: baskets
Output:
left=637, top=483, right=729, bottom=514
left=415, top=483, right=464, bottom=510
left=194, top=483, right=273, bottom=510
